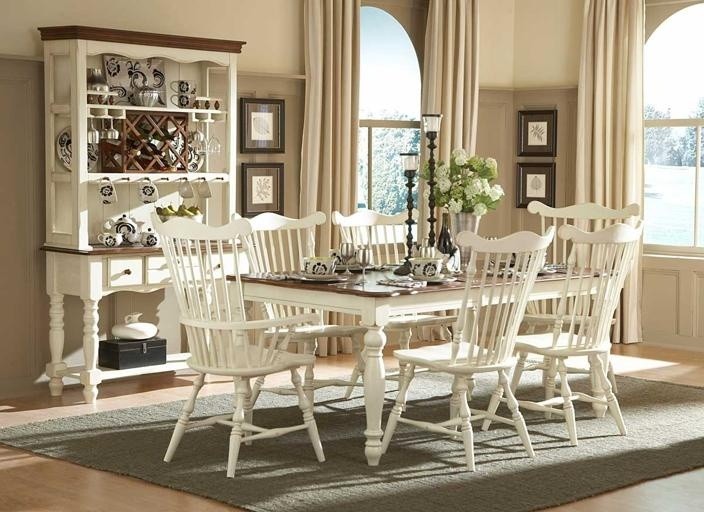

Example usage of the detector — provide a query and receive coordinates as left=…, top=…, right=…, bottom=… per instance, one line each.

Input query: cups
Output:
left=196, top=179, right=212, bottom=197
left=98, top=177, right=118, bottom=204
left=97, top=233, right=124, bottom=247
left=137, top=178, right=158, bottom=203
left=170, top=95, right=196, bottom=108
left=170, top=79, right=196, bottom=94
left=141, top=228, right=160, bottom=247
left=178, top=177, right=194, bottom=198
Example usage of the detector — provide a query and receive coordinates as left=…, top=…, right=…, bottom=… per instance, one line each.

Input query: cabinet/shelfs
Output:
left=38, top=27, right=254, bottom=184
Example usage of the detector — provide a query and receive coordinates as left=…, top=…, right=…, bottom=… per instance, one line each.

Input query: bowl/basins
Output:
left=409, top=257, right=442, bottom=275
left=304, top=256, right=336, bottom=279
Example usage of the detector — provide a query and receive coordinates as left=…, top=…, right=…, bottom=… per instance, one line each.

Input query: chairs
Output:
left=496, top=219, right=645, bottom=446
left=231, top=212, right=370, bottom=408
left=381, top=225, right=557, bottom=471
left=511, top=201, right=641, bottom=393
left=151, top=212, right=326, bottom=478
left=332, top=209, right=458, bottom=398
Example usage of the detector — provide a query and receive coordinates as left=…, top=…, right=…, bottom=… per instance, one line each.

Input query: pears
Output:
left=155, top=204, right=202, bottom=223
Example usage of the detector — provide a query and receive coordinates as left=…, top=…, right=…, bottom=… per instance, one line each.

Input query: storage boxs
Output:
left=99, top=338, right=166, bottom=369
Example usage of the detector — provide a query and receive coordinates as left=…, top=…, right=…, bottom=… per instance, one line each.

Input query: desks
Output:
left=41, top=242, right=256, bottom=404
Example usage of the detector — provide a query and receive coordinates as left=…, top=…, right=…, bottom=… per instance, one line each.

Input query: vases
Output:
left=450, top=212, right=480, bottom=282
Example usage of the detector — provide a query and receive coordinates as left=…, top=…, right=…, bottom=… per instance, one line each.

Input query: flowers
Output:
left=420, top=148, right=506, bottom=217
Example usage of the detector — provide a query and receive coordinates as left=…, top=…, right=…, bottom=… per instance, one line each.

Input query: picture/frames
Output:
left=240, top=98, right=285, bottom=153
left=516, top=162, right=556, bottom=208
left=241, top=163, right=284, bottom=217
left=517, top=109, right=557, bottom=157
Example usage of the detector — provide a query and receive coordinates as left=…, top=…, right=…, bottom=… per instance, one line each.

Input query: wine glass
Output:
left=193, top=123, right=221, bottom=154
left=420, top=247, right=435, bottom=257
left=87, top=118, right=119, bottom=144
left=341, top=243, right=355, bottom=275
left=356, top=249, right=370, bottom=284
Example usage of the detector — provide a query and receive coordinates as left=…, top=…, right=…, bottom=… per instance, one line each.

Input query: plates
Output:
left=408, top=274, right=445, bottom=281
left=427, top=274, right=458, bottom=284
left=55, top=125, right=100, bottom=172
left=300, top=275, right=349, bottom=282
left=169, top=130, right=205, bottom=172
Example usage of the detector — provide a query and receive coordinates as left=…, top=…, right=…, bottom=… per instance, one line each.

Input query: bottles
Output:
left=437, top=213, right=453, bottom=253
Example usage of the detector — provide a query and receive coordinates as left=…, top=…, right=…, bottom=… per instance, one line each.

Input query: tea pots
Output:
left=101, top=213, right=144, bottom=245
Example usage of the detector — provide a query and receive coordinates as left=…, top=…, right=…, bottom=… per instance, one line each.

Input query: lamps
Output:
left=394, top=153, right=419, bottom=275
left=423, top=115, right=445, bottom=247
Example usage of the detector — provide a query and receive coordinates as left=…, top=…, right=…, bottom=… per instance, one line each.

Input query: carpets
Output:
left=1, top=357, right=703, bottom=512
